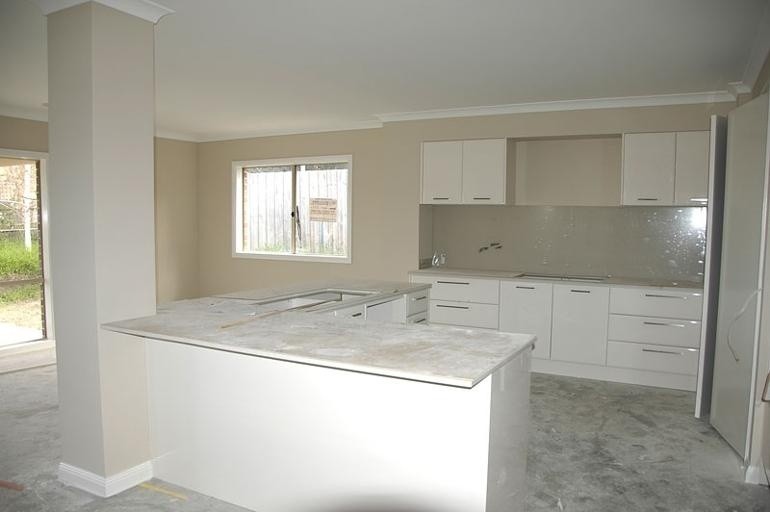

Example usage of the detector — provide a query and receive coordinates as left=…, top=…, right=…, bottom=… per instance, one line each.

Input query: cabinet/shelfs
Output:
left=608, top=285, right=701, bottom=375
left=409, top=276, right=499, bottom=329
left=420, top=138, right=507, bottom=207
left=406, top=288, right=430, bottom=326
left=498, top=282, right=608, bottom=364
left=621, top=131, right=707, bottom=206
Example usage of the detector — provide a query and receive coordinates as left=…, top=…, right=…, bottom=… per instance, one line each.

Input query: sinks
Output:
left=251, top=298, right=336, bottom=309
left=302, top=287, right=380, bottom=303
left=511, top=273, right=568, bottom=281
left=561, top=277, right=605, bottom=282
